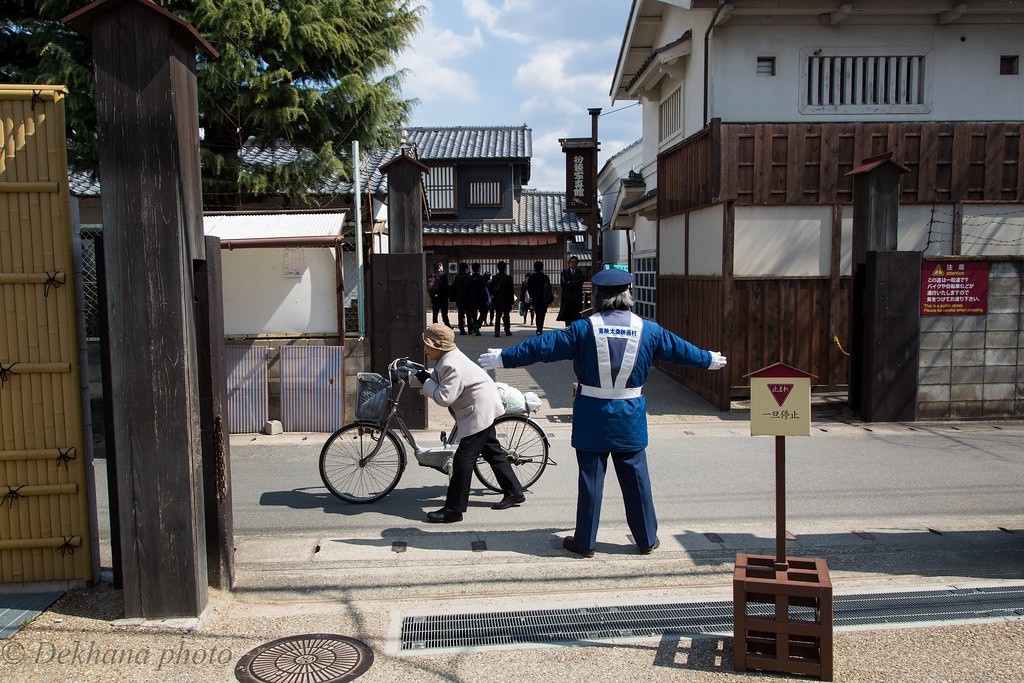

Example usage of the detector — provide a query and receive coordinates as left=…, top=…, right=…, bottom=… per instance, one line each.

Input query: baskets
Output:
left=354, top=372, right=391, bottom=422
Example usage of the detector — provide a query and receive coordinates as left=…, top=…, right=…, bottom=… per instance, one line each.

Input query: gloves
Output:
left=415, top=369, right=431, bottom=383
left=477, top=348, right=504, bottom=369
left=708, top=350, right=727, bottom=369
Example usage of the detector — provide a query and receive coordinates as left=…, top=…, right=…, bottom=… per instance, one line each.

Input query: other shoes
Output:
left=495, top=334, right=500, bottom=337
left=476, top=332, right=481, bottom=336
left=531, top=322, right=534, bottom=325
left=460, top=330, right=467, bottom=334
left=489, top=322, right=493, bottom=326
left=484, top=322, right=488, bottom=325
left=505, top=331, right=512, bottom=335
left=468, top=330, right=472, bottom=335
left=536, top=332, right=541, bottom=335
left=524, top=321, right=526, bottom=324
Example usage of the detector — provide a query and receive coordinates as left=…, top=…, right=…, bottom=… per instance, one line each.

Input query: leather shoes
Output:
left=640, top=536, right=660, bottom=553
left=428, top=508, right=463, bottom=522
left=493, top=491, right=526, bottom=508
left=562, top=535, right=594, bottom=556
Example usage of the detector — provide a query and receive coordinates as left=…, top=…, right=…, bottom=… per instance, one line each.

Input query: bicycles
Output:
left=318, top=355, right=558, bottom=504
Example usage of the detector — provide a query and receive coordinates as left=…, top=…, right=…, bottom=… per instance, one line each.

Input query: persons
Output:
left=427, top=254, right=587, bottom=341
left=406, top=323, right=528, bottom=524
left=477, top=267, right=726, bottom=557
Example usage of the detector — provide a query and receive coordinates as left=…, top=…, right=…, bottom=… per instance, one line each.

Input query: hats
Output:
left=591, top=267, right=633, bottom=291
left=421, top=322, right=456, bottom=351
